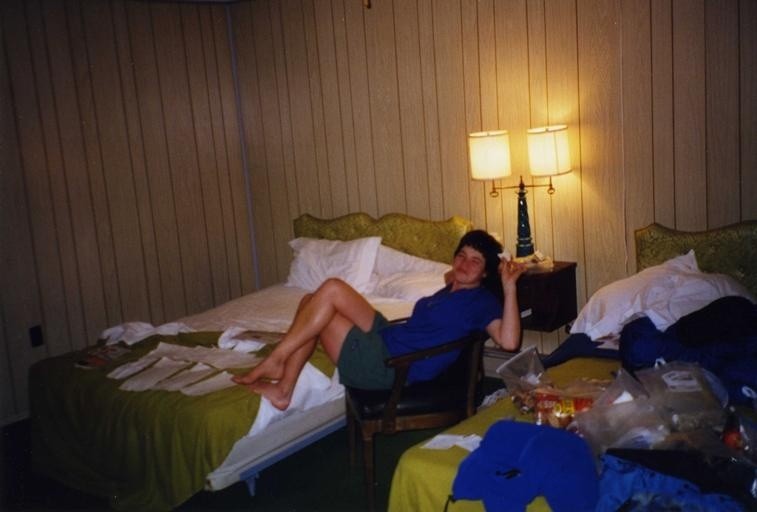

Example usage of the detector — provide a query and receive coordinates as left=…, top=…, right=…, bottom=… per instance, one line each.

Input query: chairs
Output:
left=344, top=316, right=484, bottom=511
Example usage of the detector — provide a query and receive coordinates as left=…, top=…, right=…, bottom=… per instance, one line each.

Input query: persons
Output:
left=232, top=230, right=526, bottom=417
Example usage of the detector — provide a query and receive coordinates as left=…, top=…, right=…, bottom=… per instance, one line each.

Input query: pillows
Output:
left=285, top=235, right=383, bottom=294
left=569, top=249, right=756, bottom=342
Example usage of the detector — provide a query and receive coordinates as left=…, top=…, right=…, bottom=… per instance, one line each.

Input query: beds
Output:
left=387, top=223, right=757, bottom=512
left=21, top=213, right=507, bottom=512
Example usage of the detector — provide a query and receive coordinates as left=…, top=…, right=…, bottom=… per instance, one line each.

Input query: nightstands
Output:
left=491, top=261, right=578, bottom=332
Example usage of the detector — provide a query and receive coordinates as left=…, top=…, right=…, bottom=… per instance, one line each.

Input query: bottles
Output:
left=723, top=404, right=741, bottom=450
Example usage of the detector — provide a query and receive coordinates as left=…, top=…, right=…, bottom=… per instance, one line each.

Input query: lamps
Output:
left=467, top=125, right=572, bottom=261
